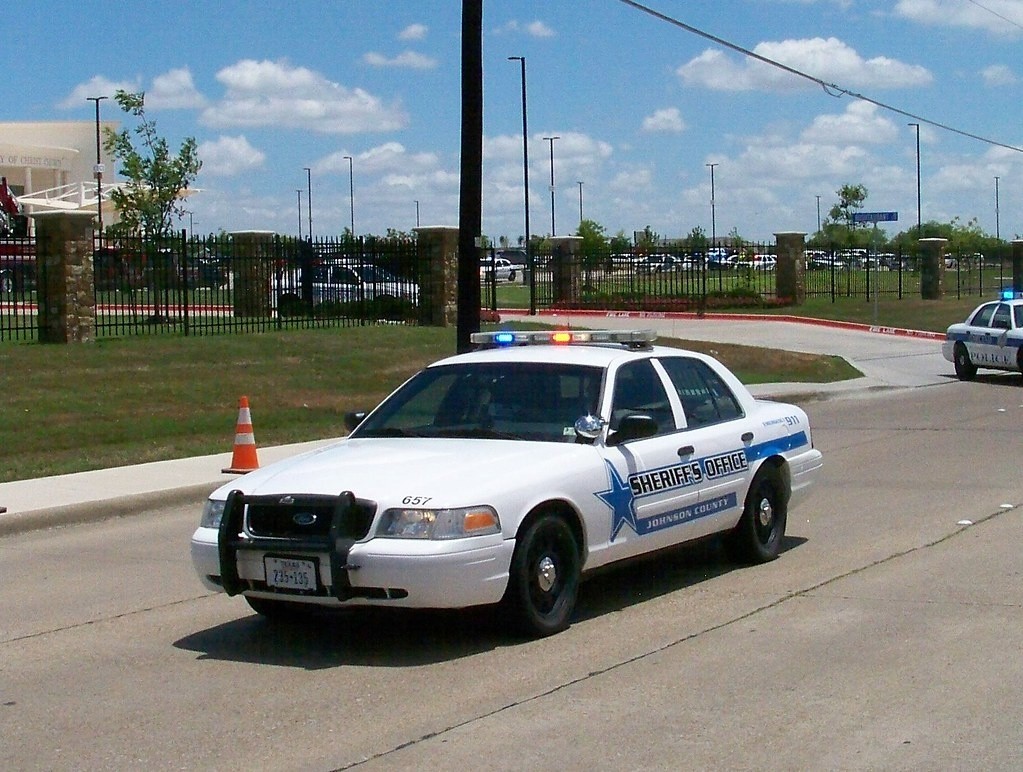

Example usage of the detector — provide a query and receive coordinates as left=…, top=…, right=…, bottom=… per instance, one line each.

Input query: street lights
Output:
left=908, top=122, right=921, bottom=242
left=542, top=136, right=561, bottom=238
left=342, top=156, right=354, bottom=238
left=413, top=200, right=422, bottom=229
left=506, top=56, right=531, bottom=285
left=706, top=163, right=719, bottom=254
left=994, top=175, right=1001, bottom=268
left=304, top=167, right=312, bottom=245
left=86, top=96, right=109, bottom=250
left=576, top=181, right=585, bottom=221
left=295, top=190, right=304, bottom=240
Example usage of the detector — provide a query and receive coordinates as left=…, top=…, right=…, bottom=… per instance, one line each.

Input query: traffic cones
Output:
left=221, top=396, right=260, bottom=474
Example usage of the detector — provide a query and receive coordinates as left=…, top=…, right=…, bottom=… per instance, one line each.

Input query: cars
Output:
left=3, top=244, right=985, bottom=305
left=941, top=290, right=1023, bottom=384
left=188, top=326, right=824, bottom=642
